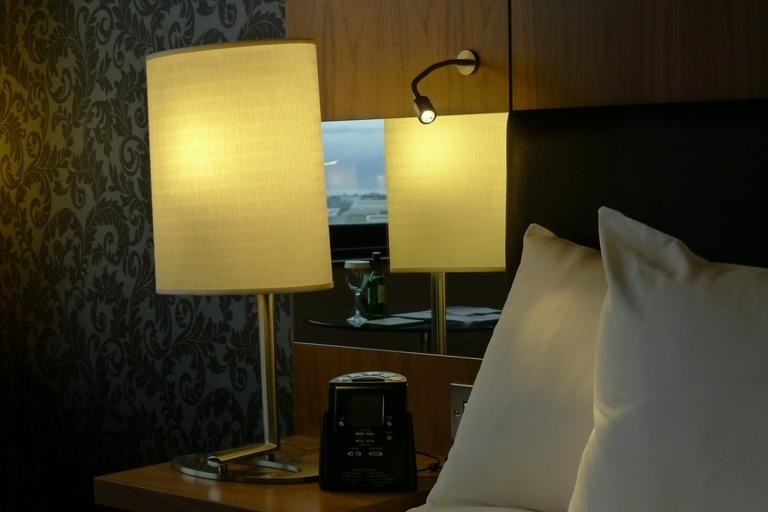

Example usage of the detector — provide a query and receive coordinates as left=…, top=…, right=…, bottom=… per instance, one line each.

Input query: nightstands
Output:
left=95, top=435, right=446, bottom=511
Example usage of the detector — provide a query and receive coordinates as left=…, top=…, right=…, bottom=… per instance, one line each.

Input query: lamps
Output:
left=385, top=113, right=510, bottom=356
left=146, top=39, right=333, bottom=484
left=411, top=50, right=477, bottom=124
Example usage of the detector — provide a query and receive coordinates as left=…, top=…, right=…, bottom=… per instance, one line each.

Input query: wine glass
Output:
left=343, top=259, right=369, bottom=327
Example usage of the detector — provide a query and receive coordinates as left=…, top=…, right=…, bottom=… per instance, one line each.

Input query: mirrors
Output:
left=293, top=120, right=507, bottom=362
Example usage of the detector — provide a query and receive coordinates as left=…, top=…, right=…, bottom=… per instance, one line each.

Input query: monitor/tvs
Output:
left=319, top=118, right=390, bottom=262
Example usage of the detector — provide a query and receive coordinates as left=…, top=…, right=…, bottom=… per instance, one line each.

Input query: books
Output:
left=364, top=304, right=502, bottom=329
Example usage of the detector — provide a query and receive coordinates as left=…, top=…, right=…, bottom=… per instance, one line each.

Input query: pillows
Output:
left=426, top=224, right=607, bottom=512
left=569, top=208, right=768, bottom=512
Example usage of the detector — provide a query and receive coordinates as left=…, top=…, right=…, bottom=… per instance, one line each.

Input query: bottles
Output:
left=366, top=252, right=392, bottom=321
